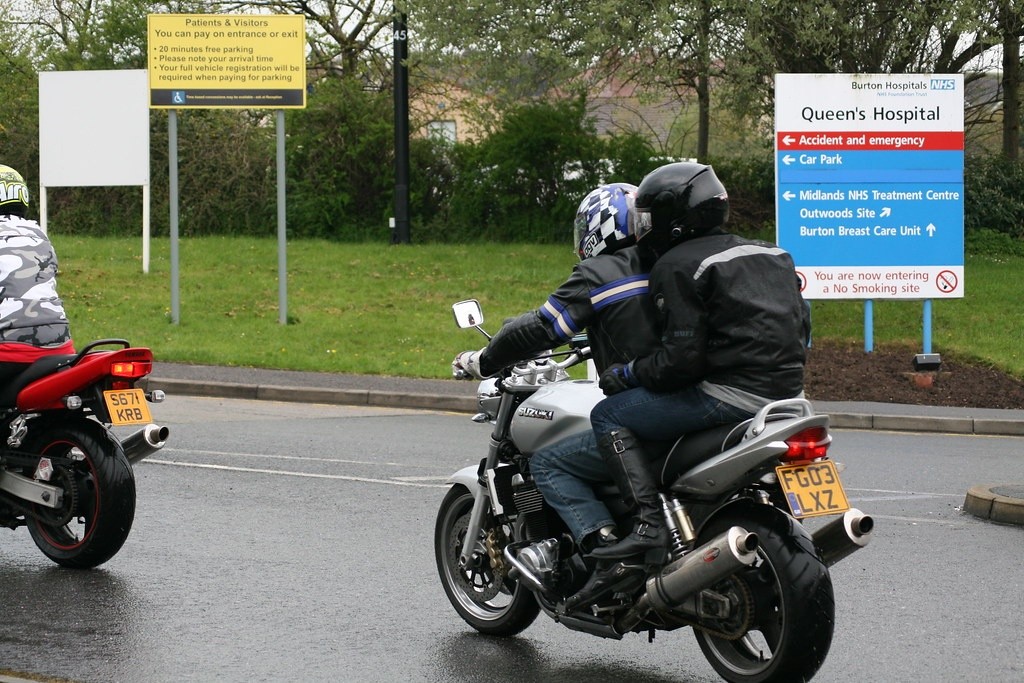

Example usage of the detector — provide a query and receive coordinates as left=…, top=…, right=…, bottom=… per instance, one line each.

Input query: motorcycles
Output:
left=1, top=338, right=170, bottom=566
left=432, top=300, right=876, bottom=683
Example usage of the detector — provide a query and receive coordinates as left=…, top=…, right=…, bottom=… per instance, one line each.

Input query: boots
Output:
left=564, top=524, right=647, bottom=613
left=588, top=425, right=673, bottom=568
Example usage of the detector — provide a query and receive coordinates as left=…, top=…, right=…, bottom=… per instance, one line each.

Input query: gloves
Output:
left=452, top=346, right=502, bottom=381
left=599, top=358, right=639, bottom=396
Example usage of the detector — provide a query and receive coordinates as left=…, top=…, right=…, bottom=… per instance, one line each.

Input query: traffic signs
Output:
left=774, top=71, right=965, bottom=301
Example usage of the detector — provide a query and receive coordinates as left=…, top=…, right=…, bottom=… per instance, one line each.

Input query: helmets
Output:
left=634, top=162, right=731, bottom=273
left=0, top=165, right=30, bottom=217
left=573, top=183, right=654, bottom=261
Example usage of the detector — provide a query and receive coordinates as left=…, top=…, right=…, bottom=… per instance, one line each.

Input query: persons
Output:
left=0, top=164, right=83, bottom=391
left=450, top=183, right=670, bottom=611
left=588, top=159, right=814, bottom=568
